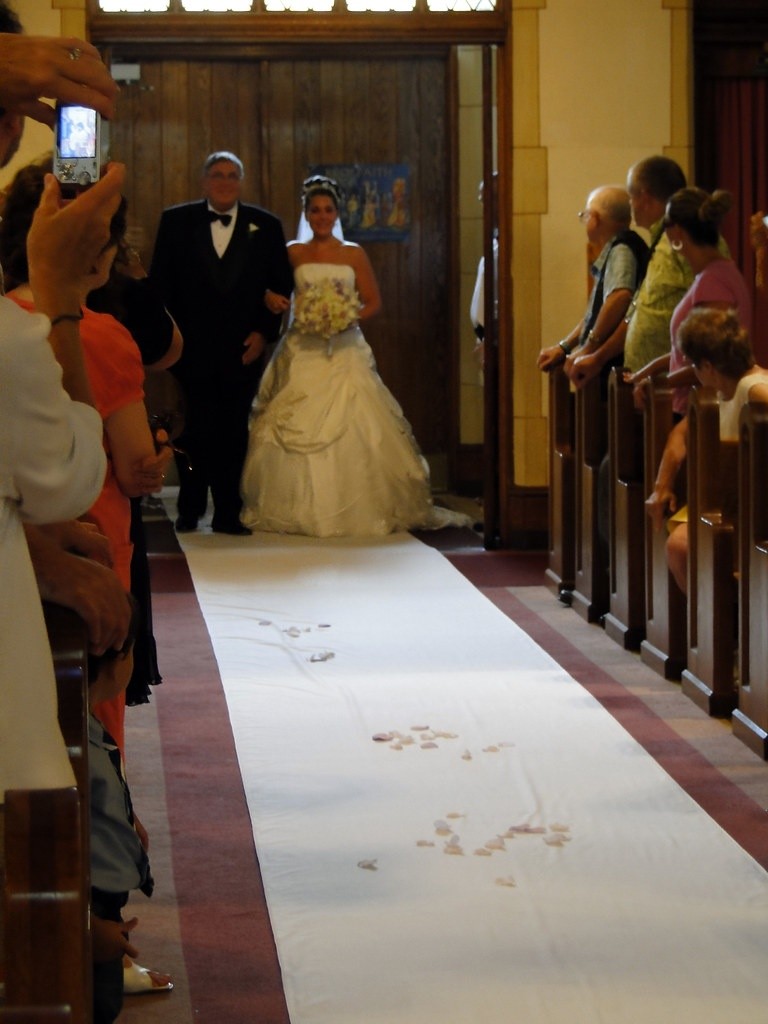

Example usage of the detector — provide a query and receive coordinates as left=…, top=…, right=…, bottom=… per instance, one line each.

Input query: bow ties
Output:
left=208, top=211, right=232, bottom=226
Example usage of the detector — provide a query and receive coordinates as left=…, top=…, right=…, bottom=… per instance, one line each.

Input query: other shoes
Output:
left=212, top=520, right=252, bottom=536
left=176, top=514, right=199, bottom=533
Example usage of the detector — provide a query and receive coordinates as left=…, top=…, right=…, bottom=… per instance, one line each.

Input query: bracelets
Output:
left=588, top=330, right=601, bottom=343
left=52, top=307, right=85, bottom=327
left=558, top=341, right=570, bottom=353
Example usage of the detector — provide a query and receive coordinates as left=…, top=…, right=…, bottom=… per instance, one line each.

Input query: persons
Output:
left=470, top=170, right=499, bottom=529
left=150, top=150, right=295, bottom=536
left=241, top=176, right=475, bottom=539
left=535, top=152, right=768, bottom=595
left=0, top=0, right=182, bottom=1022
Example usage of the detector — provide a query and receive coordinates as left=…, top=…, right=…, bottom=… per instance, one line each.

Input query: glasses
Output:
left=579, top=211, right=591, bottom=223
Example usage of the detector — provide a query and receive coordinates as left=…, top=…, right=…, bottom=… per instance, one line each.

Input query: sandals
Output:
left=123, top=954, right=174, bottom=993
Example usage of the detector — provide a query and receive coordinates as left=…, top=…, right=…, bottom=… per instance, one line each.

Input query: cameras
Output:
left=52, top=98, right=103, bottom=187
